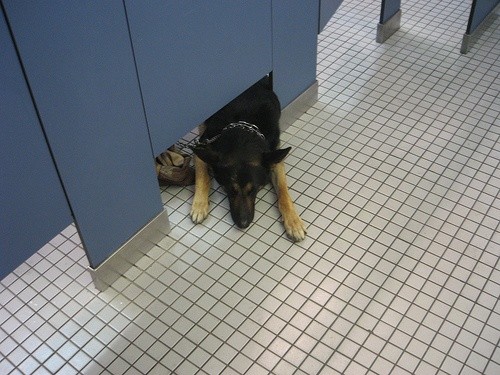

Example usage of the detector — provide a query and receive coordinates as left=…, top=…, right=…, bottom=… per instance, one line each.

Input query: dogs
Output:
left=188, top=75, right=307, bottom=243
